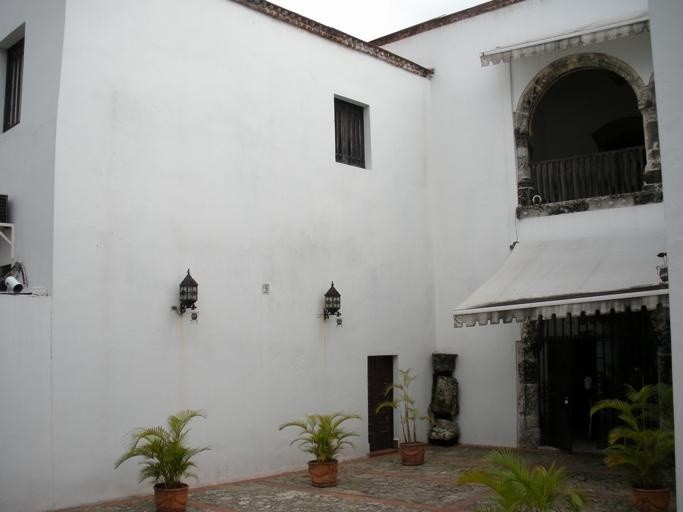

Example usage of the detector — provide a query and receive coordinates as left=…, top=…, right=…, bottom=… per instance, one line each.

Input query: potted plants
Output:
left=114, top=408, right=212, bottom=512
left=375, top=369, right=438, bottom=466
left=278, top=411, right=364, bottom=487
left=590, top=384, right=674, bottom=512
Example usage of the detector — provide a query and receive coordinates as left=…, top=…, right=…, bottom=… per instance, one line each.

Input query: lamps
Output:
left=170, top=269, right=198, bottom=321
left=324, top=281, right=343, bottom=325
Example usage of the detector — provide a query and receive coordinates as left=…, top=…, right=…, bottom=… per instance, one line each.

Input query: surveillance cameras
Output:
left=5, top=276, right=23, bottom=292
